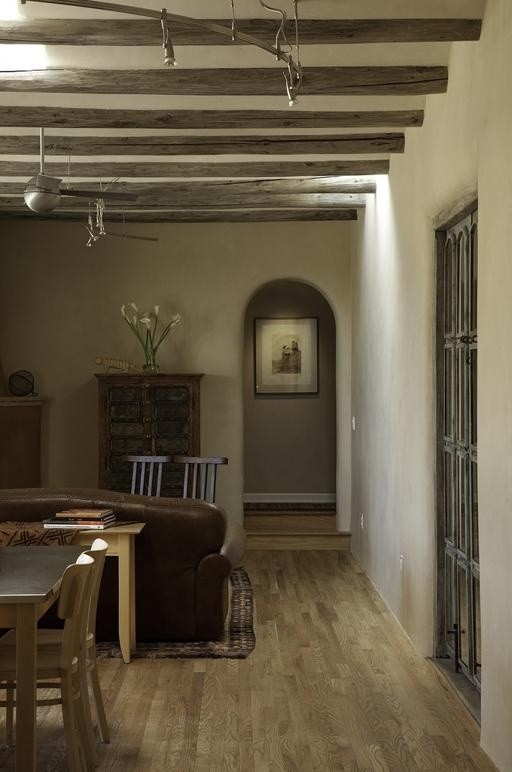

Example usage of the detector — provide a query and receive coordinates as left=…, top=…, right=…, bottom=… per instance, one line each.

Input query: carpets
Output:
left=85, top=563, right=256, bottom=659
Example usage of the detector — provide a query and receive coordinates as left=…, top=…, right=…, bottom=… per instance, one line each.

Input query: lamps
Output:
left=1, top=0, right=299, bottom=108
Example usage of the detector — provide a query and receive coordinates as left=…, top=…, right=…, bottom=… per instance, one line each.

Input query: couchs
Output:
left=0, top=487, right=247, bottom=641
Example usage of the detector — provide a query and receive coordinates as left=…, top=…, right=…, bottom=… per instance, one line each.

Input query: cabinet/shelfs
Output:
left=0, top=396, right=48, bottom=489
left=94, top=372, right=205, bottom=500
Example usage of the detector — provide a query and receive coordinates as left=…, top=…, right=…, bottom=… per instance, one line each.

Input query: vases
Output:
left=143, top=349, right=160, bottom=375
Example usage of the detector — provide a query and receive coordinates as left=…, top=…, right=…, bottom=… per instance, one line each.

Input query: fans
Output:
left=23, top=126, right=138, bottom=212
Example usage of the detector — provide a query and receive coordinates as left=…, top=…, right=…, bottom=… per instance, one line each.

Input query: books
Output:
left=43, top=509, right=116, bottom=529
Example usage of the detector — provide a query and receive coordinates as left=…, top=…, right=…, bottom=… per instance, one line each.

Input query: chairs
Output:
left=173, top=455, right=229, bottom=504
left=0, top=538, right=110, bottom=770
left=126, top=455, right=172, bottom=497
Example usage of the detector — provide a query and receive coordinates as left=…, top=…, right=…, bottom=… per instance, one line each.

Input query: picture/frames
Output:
left=253, top=316, right=320, bottom=396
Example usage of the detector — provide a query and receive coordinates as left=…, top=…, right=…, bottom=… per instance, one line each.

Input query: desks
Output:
left=0, top=520, right=148, bottom=664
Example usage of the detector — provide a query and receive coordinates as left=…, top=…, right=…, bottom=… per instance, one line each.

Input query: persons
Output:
left=282, top=346, right=292, bottom=371
left=292, top=341, right=298, bottom=360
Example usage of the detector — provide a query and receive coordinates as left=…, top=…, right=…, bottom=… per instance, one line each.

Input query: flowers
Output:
left=120, top=301, right=182, bottom=367
